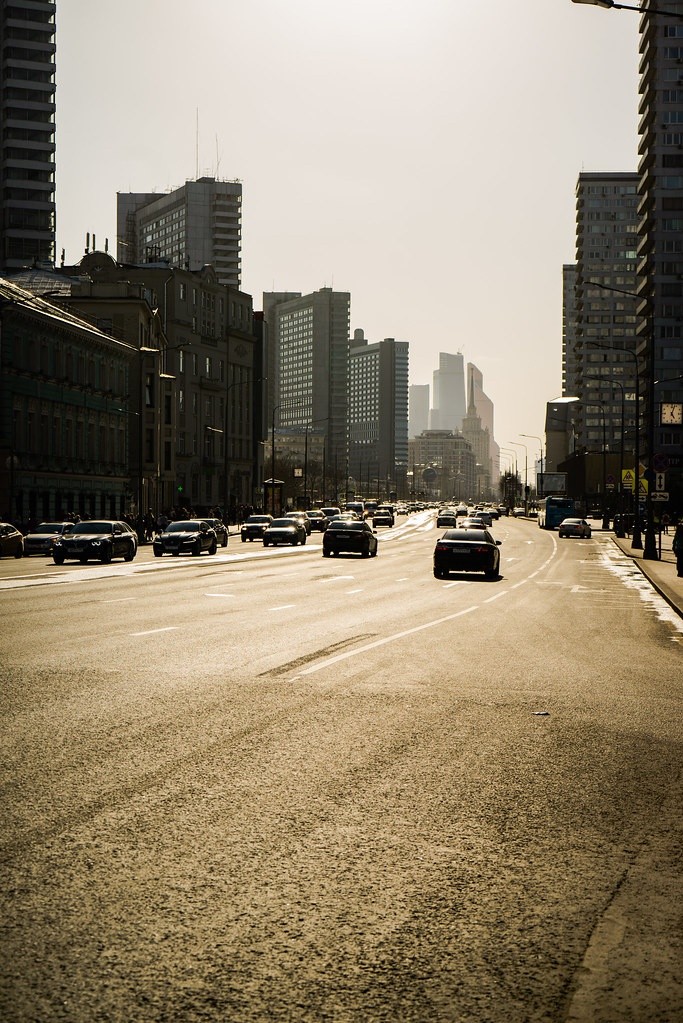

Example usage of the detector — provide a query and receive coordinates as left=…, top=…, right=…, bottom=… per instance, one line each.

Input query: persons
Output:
left=63, top=503, right=313, bottom=544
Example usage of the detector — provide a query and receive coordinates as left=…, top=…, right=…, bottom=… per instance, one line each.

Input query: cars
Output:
left=559, top=518, right=592, bottom=539
left=23, top=522, right=75, bottom=558
left=52, top=519, right=138, bottom=565
left=436, top=500, right=526, bottom=530
left=433, top=528, right=502, bottom=580
left=613, top=514, right=647, bottom=535
left=0, top=522, right=24, bottom=559
left=190, top=518, right=228, bottom=547
left=153, top=520, right=218, bottom=557
left=240, top=501, right=436, bottom=558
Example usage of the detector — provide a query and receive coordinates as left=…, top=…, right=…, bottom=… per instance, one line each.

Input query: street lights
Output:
left=568, top=402, right=609, bottom=529
left=303, top=418, right=332, bottom=512
left=549, top=416, right=576, bottom=456
left=222, top=377, right=268, bottom=526
left=495, top=434, right=543, bottom=517
left=323, top=432, right=343, bottom=508
left=272, top=401, right=303, bottom=519
left=583, top=281, right=657, bottom=560
left=137, top=342, right=191, bottom=544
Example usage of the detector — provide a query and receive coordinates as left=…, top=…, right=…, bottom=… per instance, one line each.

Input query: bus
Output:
left=537, top=496, right=574, bottom=531
